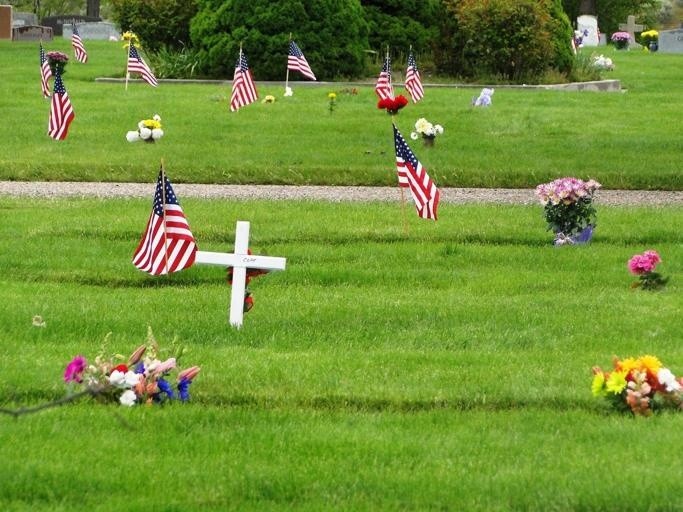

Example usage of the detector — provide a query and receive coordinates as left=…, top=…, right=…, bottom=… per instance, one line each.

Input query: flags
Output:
left=72, top=21, right=87, bottom=63
left=286, top=40, right=317, bottom=81
left=230, top=49, right=257, bottom=112
left=374, top=56, right=395, bottom=102
left=39, top=43, right=51, bottom=98
left=132, top=163, right=198, bottom=276
left=47, top=67, right=74, bottom=142
left=393, top=128, right=439, bottom=221
left=404, top=50, right=425, bottom=103
left=126, top=41, right=157, bottom=87
